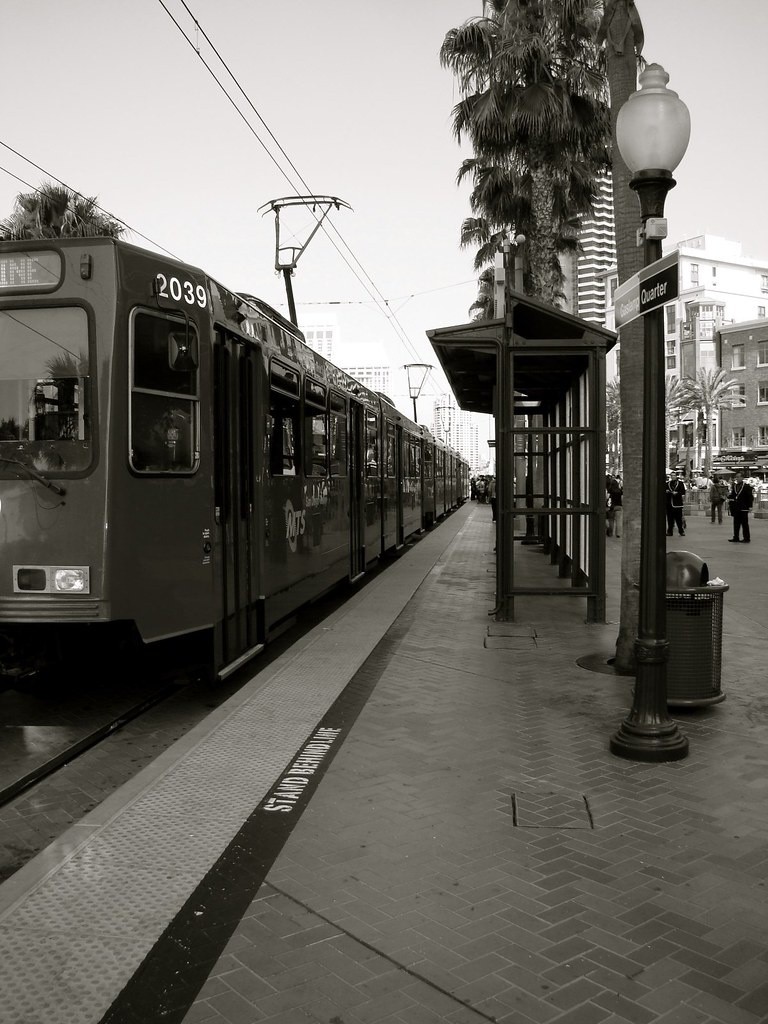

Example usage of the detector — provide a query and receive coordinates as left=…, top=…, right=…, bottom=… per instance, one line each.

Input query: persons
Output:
left=605, top=473, right=621, bottom=537
left=728, top=471, right=754, bottom=543
left=665, top=469, right=716, bottom=504
left=470, top=475, right=497, bottom=523
left=718, top=475, right=735, bottom=499
left=607, top=479, right=623, bottom=539
left=709, top=477, right=724, bottom=525
left=665, top=472, right=686, bottom=536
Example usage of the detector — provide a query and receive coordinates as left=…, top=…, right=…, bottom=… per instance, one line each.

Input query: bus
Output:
left=1, top=232, right=476, bottom=688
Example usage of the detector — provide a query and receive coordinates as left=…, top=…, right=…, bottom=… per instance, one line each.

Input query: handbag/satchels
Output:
left=719, top=494, right=727, bottom=502
left=607, top=498, right=612, bottom=506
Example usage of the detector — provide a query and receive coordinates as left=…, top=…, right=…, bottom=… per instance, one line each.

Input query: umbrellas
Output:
left=713, top=469, right=736, bottom=479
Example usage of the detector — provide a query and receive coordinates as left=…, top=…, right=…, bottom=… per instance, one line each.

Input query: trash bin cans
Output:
left=664, top=550, right=729, bottom=707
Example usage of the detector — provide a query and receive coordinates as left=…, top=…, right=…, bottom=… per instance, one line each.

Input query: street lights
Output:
left=606, top=60, right=691, bottom=764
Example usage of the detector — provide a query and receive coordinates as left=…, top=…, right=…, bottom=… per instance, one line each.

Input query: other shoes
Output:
left=607, top=528, right=612, bottom=537
left=739, top=539, right=751, bottom=543
left=616, top=535, right=620, bottom=538
left=728, top=538, right=740, bottom=542
left=709, top=521, right=715, bottom=524
left=679, top=532, right=685, bottom=536
left=666, top=532, right=673, bottom=536
